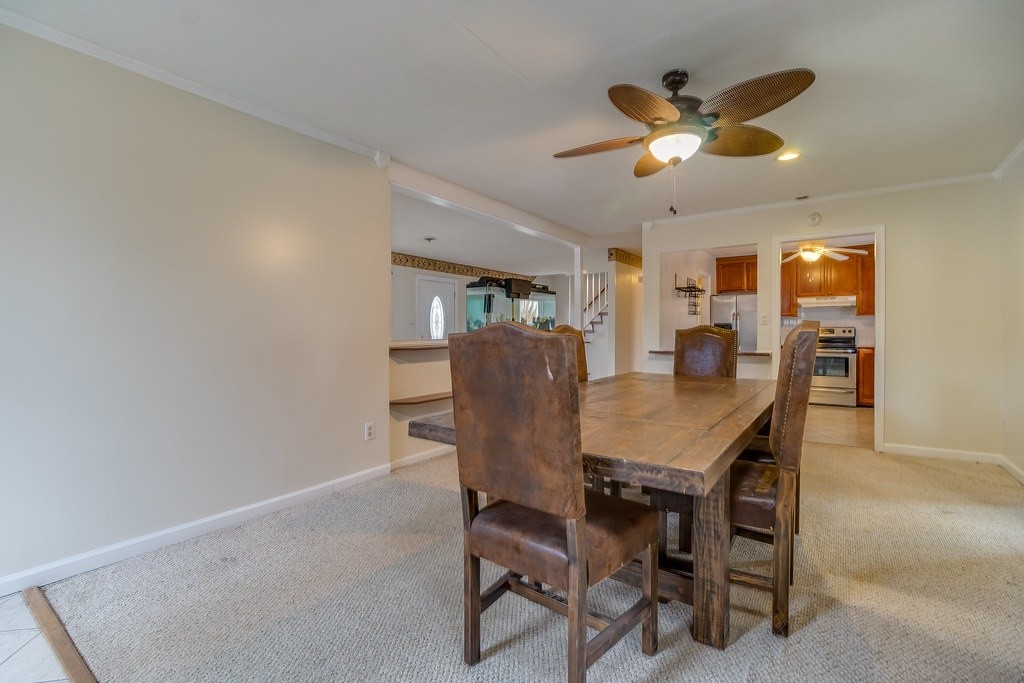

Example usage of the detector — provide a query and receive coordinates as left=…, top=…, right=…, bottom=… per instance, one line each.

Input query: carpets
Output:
left=37, top=438, right=1024, bottom=683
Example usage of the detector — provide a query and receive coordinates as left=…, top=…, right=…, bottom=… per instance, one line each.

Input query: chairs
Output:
left=448, top=321, right=659, bottom=682
left=547, top=324, right=623, bottom=499
left=641, top=321, right=821, bottom=639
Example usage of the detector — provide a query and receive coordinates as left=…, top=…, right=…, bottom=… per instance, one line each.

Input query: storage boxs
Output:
left=466, top=276, right=556, bottom=333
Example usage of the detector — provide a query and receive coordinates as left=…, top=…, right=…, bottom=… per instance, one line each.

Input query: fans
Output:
left=553, top=67, right=817, bottom=179
left=781, top=240, right=868, bottom=263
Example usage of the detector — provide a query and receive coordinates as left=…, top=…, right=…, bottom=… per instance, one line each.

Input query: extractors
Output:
left=797, top=295, right=856, bottom=321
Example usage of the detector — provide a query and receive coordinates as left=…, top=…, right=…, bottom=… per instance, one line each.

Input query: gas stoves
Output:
left=816, top=327, right=856, bottom=348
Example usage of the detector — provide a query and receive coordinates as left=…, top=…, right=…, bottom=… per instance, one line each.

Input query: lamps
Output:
left=643, top=120, right=709, bottom=166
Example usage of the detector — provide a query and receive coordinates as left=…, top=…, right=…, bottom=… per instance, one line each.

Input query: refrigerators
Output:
left=710, top=293, right=757, bottom=352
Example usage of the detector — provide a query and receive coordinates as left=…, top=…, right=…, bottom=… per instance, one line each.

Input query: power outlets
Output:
left=364, top=422, right=374, bottom=440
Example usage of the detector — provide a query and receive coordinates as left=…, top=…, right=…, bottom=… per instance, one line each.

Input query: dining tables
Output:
left=408, top=371, right=778, bottom=650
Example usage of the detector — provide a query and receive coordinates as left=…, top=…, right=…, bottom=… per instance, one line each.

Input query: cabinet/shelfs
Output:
left=676, top=285, right=706, bottom=316
left=797, top=246, right=857, bottom=307
left=781, top=252, right=799, bottom=317
left=857, top=347, right=874, bottom=407
left=715, top=255, right=757, bottom=294
left=857, top=244, right=874, bottom=316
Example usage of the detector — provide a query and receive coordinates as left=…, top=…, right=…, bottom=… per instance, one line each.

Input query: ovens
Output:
left=809, top=349, right=856, bottom=407
